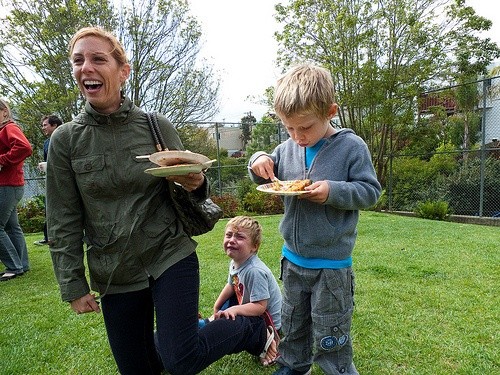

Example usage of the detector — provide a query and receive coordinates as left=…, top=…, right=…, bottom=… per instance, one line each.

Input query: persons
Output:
left=153, top=216, right=282, bottom=357
left=33, top=116, right=62, bottom=246
left=247, top=64, right=382, bottom=375
left=0, top=100, right=32, bottom=277
left=45, top=28, right=212, bottom=375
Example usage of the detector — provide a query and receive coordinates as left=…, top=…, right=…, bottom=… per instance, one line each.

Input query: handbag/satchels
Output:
left=146, top=112, right=223, bottom=235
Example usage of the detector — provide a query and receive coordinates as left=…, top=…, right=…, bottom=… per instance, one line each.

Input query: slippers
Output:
left=258, top=310, right=281, bottom=367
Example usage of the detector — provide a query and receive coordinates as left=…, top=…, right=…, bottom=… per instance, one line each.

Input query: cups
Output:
left=38, top=161, right=47, bottom=173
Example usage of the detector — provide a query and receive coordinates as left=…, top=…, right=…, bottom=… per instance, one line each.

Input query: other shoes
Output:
left=39, top=239, right=48, bottom=243
left=0, top=272, right=23, bottom=280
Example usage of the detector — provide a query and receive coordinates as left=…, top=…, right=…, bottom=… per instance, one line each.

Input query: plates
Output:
left=256, top=179, right=310, bottom=195
left=149, top=151, right=212, bottom=170
left=144, top=164, right=202, bottom=177
